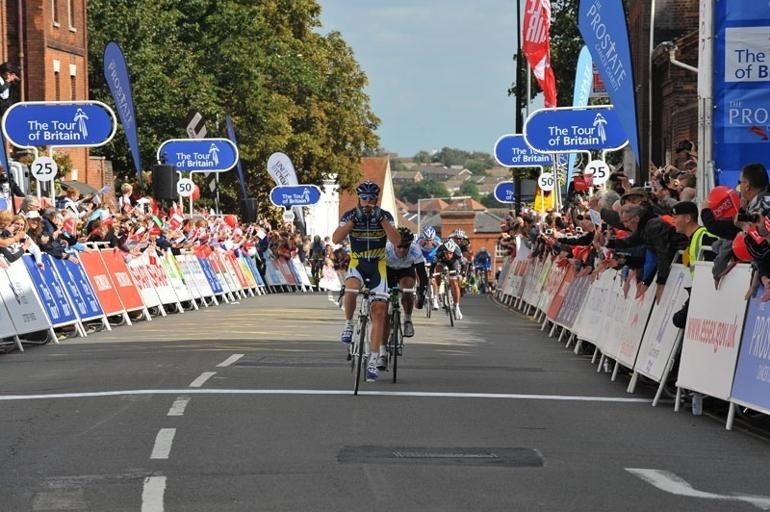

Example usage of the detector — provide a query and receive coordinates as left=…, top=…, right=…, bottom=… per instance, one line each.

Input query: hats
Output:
left=25, top=211, right=41, bottom=220
left=620, top=187, right=647, bottom=205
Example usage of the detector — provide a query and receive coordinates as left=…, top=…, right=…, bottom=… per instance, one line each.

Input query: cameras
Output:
left=14, top=75, right=21, bottom=83
left=576, top=227, right=583, bottom=232
left=546, top=229, right=553, bottom=234
left=738, top=213, right=758, bottom=223
left=20, top=239, right=26, bottom=242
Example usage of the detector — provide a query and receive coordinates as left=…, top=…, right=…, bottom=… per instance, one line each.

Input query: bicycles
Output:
left=424, top=261, right=491, bottom=328
left=337, top=284, right=394, bottom=395
left=386, top=285, right=428, bottom=384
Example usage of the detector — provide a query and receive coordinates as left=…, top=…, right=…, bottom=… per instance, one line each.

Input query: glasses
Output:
left=359, top=194, right=377, bottom=200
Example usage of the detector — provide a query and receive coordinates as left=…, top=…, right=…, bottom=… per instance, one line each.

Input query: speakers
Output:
left=240, top=198, right=257, bottom=223
left=153, top=165, right=177, bottom=201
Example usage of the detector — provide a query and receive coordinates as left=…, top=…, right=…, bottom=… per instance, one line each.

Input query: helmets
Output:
left=398, top=227, right=414, bottom=245
left=356, top=181, right=380, bottom=194
left=423, top=225, right=464, bottom=252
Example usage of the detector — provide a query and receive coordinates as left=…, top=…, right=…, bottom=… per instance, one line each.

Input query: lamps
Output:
left=658, top=38, right=697, bottom=74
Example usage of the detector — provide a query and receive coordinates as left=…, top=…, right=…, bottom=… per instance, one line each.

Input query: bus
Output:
left=8, top=160, right=30, bottom=196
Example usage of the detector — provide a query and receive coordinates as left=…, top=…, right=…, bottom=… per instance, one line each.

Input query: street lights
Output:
left=416, top=195, right=472, bottom=239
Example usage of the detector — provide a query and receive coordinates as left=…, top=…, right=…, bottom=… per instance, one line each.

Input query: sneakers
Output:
left=342, top=327, right=352, bottom=342
left=454, top=306, right=462, bottom=320
left=433, top=298, right=439, bottom=309
left=367, top=352, right=388, bottom=379
left=404, top=321, right=413, bottom=337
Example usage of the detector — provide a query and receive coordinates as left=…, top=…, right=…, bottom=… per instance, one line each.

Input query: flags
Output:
left=226, top=117, right=244, bottom=186
left=265, top=151, right=302, bottom=224
left=575, top=0, right=640, bottom=166
left=103, top=41, right=142, bottom=179
left=522, top=0, right=558, bottom=112
left=184, top=108, right=219, bottom=201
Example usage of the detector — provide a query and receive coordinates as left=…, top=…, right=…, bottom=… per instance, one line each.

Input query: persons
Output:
left=302, top=234, right=351, bottom=291
left=0, top=61, right=21, bottom=119
left=0, top=173, right=301, bottom=352
left=496, top=139, right=769, bottom=385
left=376, top=227, right=429, bottom=369
left=415, top=225, right=492, bottom=320
left=333, top=180, right=401, bottom=378
